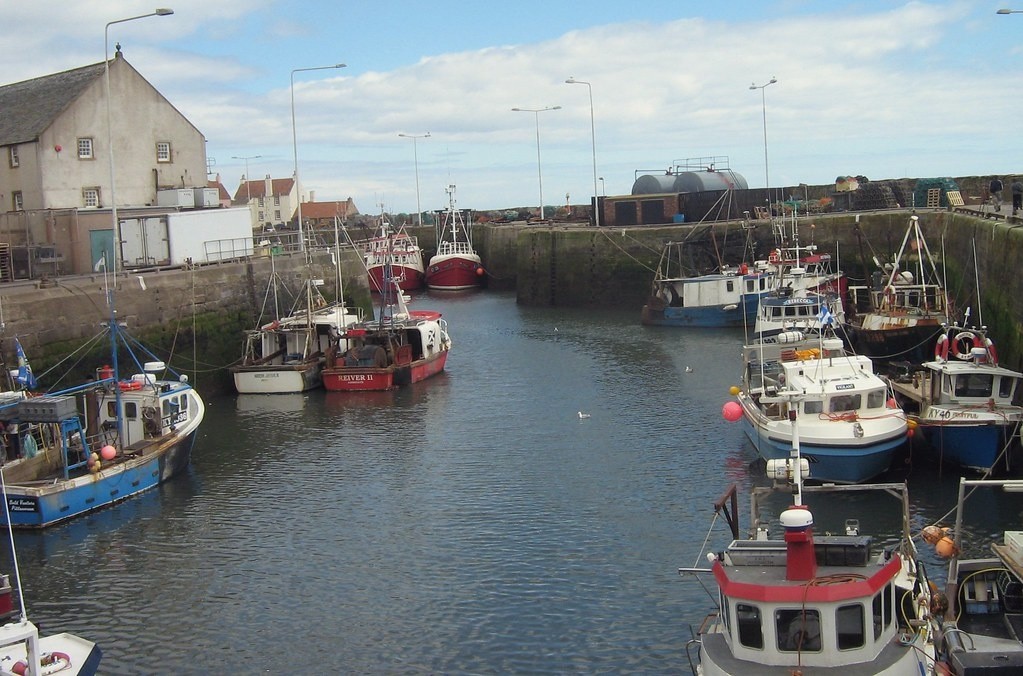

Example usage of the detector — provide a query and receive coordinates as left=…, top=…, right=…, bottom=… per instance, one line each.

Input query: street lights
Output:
left=231, top=156, right=260, bottom=203
left=511, top=106, right=563, bottom=221
left=565, top=76, right=600, bottom=228
left=749, top=75, right=778, bottom=219
left=599, top=177, right=605, bottom=197
left=104, top=7, right=176, bottom=276
left=291, top=64, right=348, bottom=251
left=397, top=131, right=432, bottom=226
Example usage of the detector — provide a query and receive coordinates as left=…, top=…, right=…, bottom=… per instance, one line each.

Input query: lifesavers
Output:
left=935, top=333, right=948, bottom=365
left=118, top=381, right=142, bottom=391
left=952, top=332, right=980, bottom=361
left=11, top=651, right=70, bottom=676
left=884, top=285, right=896, bottom=304
left=980, top=337, right=999, bottom=367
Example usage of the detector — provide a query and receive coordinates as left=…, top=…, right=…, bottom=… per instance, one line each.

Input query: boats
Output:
left=426, top=184, right=483, bottom=291
left=675, top=470, right=1023, bottom=676
left=228, top=250, right=365, bottom=394
left=365, top=200, right=426, bottom=292
left=874, top=233, right=1023, bottom=476
left=641, top=183, right=963, bottom=374
left=736, top=269, right=909, bottom=486
left=320, top=204, right=452, bottom=391
left=1, top=288, right=201, bottom=531
